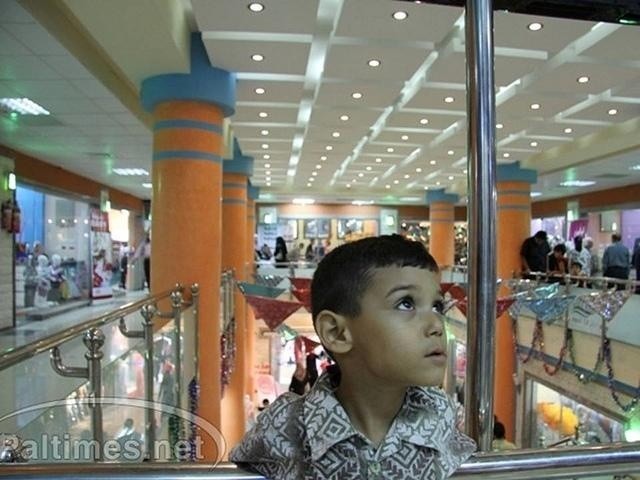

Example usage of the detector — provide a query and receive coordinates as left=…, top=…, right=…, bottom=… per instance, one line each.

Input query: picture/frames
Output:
left=277, top=219, right=299, bottom=240
left=337, top=218, right=364, bottom=240
left=303, top=218, right=331, bottom=239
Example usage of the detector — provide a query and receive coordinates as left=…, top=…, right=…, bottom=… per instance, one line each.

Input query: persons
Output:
left=306, top=349, right=324, bottom=389
left=48, top=253, right=67, bottom=304
left=23, top=255, right=38, bottom=308
left=141, top=236, right=150, bottom=288
left=289, top=365, right=310, bottom=396
left=319, top=356, right=332, bottom=372
left=253, top=233, right=331, bottom=268
left=492, top=414, right=508, bottom=451
left=117, top=252, right=130, bottom=290
left=35, top=255, right=56, bottom=309
left=115, top=418, right=140, bottom=442
left=258, top=399, right=269, bottom=410
left=157, top=361, right=177, bottom=421
left=519, top=228, right=640, bottom=294
left=230, top=232, right=480, bottom=480
